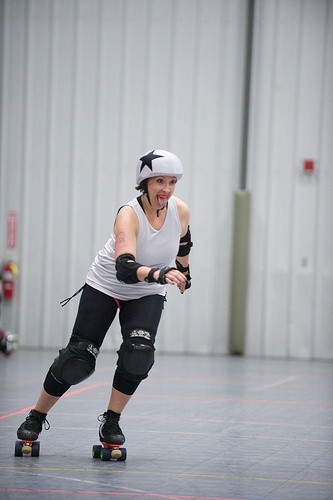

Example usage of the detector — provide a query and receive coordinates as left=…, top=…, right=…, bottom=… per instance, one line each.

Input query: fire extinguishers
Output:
left=1, top=257, right=18, bottom=302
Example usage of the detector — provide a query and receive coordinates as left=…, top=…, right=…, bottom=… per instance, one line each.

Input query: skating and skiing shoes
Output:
left=15, top=408, right=48, bottom=457
left=91, top=410, right=127, bottom=462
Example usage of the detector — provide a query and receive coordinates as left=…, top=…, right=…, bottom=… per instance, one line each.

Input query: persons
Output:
left=15, top=149, right=193, bottom=447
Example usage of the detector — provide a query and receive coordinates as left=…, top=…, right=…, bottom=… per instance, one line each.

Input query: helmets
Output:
left=136, top=149, right=183, bottom=187
